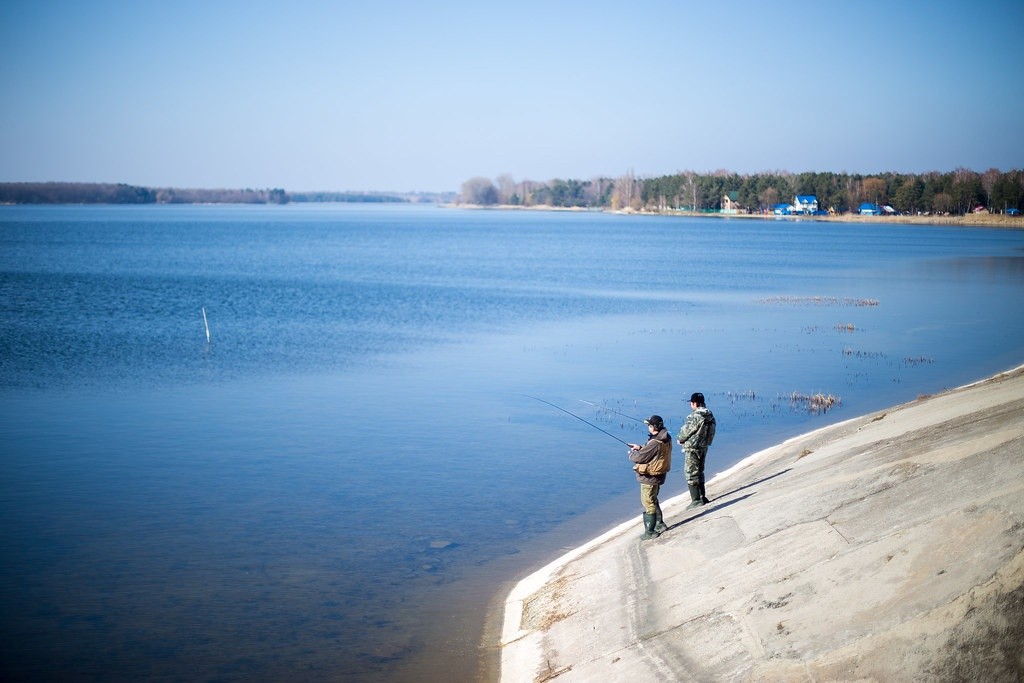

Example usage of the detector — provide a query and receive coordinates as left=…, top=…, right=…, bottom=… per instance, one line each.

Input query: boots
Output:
left=686, top=482, right=710, bottom=510
left=640, top=511, right=668, bottom=541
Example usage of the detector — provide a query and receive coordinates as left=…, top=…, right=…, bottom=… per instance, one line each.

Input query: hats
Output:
left=686, top=392, right=706, bottom=404
left=643, top=414, right=664, bottom=430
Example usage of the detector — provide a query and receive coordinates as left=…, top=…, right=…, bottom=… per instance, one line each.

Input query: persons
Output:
left=628, top=415, right=673, bottom=541
left=676, top=392, right=717, bottom=511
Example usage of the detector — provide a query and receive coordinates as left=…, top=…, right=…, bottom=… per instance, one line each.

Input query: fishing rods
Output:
left=518, top=393, right=632, bottom=448
left=578, top=398, right=679, bottom=441
